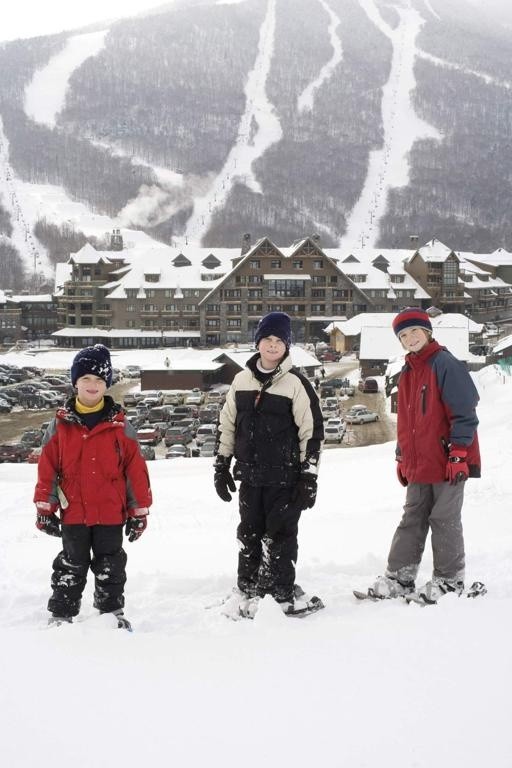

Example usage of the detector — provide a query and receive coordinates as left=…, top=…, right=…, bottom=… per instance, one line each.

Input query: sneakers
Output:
left=114, top=615, right=134, bottom=632
left=368, top=575, right=415, bottom=599
left=409, top=578, right=465, bottom=608
left=274, top=595, right=310, bottom=614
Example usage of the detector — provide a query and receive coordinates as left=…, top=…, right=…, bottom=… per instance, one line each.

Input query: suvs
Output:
left=0, top=442, right=33, bottom=463
left=314, top=347, right=341, bottom=356
left=358, top=378, right=379, bottom=393
left=41, top=421, right=50, bottom=434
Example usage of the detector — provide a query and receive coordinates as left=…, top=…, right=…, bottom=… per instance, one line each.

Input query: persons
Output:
left=213, top=311, right=325, bottom=616
left=34, top=343, right=153, bottom=632
left=350, top=306, right=488, bottom=603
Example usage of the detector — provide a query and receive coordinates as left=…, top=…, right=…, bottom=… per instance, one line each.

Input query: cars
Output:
left=320, top=378, right=347, bottom=443
left=111, top=367, right=121, bottom=384
left=122, top=365, right=144, bottom=378
left=484, top=321, right=496, bottom=330
left=317, top=352, right=340, bottom=362
left=340, top=384, right=356, bottom=397
left=29, top=447, right=43, bottom=463
left=123, top=388, right=226, bottom=460
left=20, top=428, right=44, bottom=447
left=345, top=410, right=379, bottom=424
left=348, top=405, right=368, bottom=410
left=1, top=363, right=79, bottom=413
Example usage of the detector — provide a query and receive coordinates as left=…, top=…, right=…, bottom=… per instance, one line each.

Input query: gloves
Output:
left=36, top=512, right=63, bottom=539
left=290, top=473, right=318, bottom=512
left=395, top=455, right=408, bottom=487
left=124, top=516, right=147, bottom=542
left=212, top=462, right=238, bottom=504
left=442, top=443, right=469, bottom=487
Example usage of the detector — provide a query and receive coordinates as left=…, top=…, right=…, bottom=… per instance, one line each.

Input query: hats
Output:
left=254, top=311, right=292, bottom=350
left=71, top=345, right=113, bottom=389
left=392, top=307, right=433, bottom=339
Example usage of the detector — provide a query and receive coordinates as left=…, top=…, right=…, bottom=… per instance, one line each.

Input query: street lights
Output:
left=464, top=310, right=472, bottom=329
left=38, top=330, right=41, bottom=348
left=161, top=328, right=165, bottom=347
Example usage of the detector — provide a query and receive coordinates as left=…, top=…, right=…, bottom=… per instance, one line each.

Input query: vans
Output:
left=17, top=340, right=35, bottom=350
left=468, top=344, right=493, bottom=356
